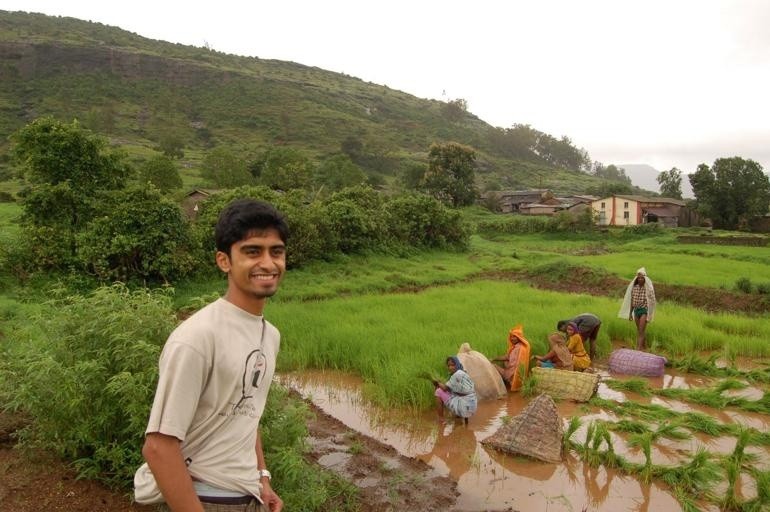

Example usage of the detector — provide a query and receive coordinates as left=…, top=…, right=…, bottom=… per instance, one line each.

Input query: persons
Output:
left=132, top=198, right=295, bottom=512
left=488, top=323, right=530, bottom=392
left=530, top=332, right=574, bottom=371
left=557, top=313, right=601, bottom=361
left=456, top=342, right=508, bottom=401
left=431, top=356, right=477, bottom=426
left=565, top=322, right=592, bottom=372
left=629, top=267, right=656, bottom=351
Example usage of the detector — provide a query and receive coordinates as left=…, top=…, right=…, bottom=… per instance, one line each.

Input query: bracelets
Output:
left=259, top=468, right=272, bottom=480
